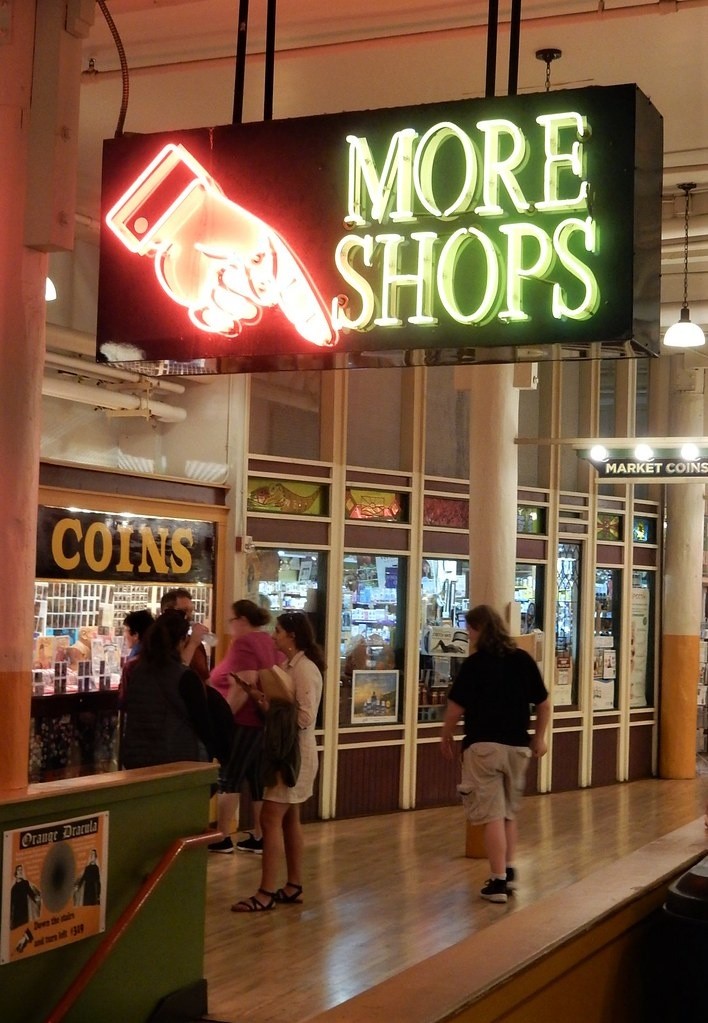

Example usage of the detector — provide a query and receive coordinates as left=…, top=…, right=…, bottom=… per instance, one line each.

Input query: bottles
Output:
left=190, top=621, right=218, bottom=647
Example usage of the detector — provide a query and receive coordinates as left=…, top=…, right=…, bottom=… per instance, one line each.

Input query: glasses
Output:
left=226, top=616, right=242, bottom=624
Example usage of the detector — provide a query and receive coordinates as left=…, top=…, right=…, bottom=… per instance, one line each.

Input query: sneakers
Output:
left=236, top=831, right=264, bottom=854
left=506, top=867, right=518, bottom=890
left=207, top=837, right=234, bottom=852
left=479, top=878, right=507, bottom=902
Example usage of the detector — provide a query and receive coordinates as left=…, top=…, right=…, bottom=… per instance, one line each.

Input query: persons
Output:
left=207, top=599, right=325, bottom=913
left=440, top=605, right=553, bottom=904
left=114, top=588, right=210, bottom=772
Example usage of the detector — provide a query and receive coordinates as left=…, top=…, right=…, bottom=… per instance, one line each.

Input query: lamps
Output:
left=664, top=183, right=705, bottom=348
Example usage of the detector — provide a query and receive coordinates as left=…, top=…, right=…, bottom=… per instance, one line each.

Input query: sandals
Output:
left=230, top=888, right=278, bottom=912
left=274, top=882, right=304, bottom=904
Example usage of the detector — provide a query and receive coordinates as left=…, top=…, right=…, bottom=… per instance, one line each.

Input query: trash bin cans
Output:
left=660, top=854, right=708, bottom=1023
